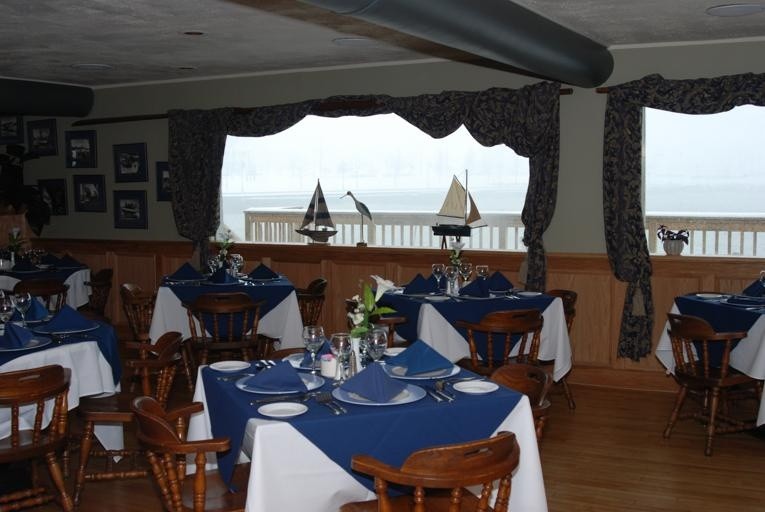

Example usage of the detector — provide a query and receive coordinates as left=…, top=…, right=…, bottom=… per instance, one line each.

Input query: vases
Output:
left=662, top=239, right=686, bottom=257
left=9, top=252, right=16, bottom=266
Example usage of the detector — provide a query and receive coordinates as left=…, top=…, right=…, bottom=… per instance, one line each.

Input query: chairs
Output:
left=663, top=310, right=765, bottom=456
left=67, top=330, right=183, bottom=505
left=2, top=362, right=75, bottom=512
left=347, top=266, right=579, bottom=427
left=121, top=270, right=327, bottom=398
left=0, top=269, right=115, bottom=322
left=134, top=390, right=228, bottom=507
left=341, top=429, right=520, bottom=510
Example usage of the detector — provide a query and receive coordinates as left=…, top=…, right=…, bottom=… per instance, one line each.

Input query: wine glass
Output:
left=366, top=329, right=389, bottom=362
left=328, top=332, right=353, bottom=387
left=302, top=324, right=326, bottom=375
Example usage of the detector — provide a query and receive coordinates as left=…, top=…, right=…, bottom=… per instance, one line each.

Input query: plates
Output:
left=692, top=290, right=765, bottom=312
left=209, top=360, right=251, bottom=373
left=452, top=381, right=500, bottom=396
left=234, top=337, right=461, bottom=405
left=254, top=401, right=309, bottom=418
left=388, top=262, right=541, bottom=302
left=1, top=228, right=103, bottom=355
left=165, top=251, right=282, bottom=288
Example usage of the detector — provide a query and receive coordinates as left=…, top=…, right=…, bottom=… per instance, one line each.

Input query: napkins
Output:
left=245, top=360, right=309, bottom=392
left=314, top=338, right=336, bottom=359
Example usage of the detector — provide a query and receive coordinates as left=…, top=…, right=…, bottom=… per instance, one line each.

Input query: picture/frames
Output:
left=0, top=113, right=174, bottom=230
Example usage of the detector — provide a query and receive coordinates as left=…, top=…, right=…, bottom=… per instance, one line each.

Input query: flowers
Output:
left=656, top=223, right=691, bottom=245
left=3, top=221, right=29, bottom=252
left=445, top=232, right=468, bottom=271
left=210, top=221, right=238, bottom=250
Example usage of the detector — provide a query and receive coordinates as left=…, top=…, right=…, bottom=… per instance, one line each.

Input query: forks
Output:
left=322, top=390, right=348, bottom=415
left=311, top=393, right=342, bottom=417
